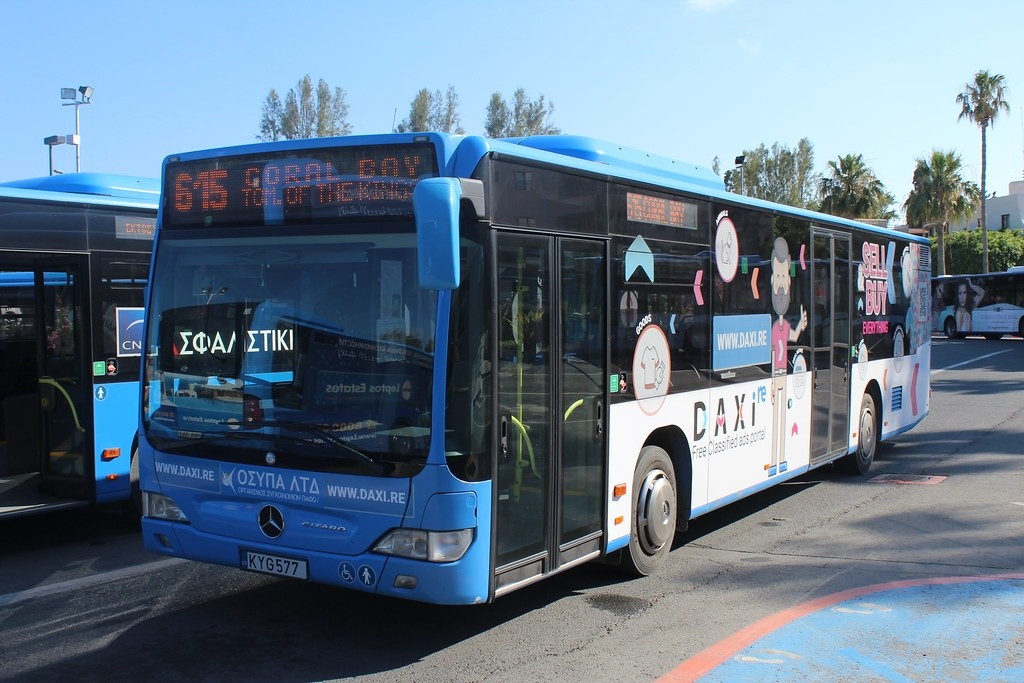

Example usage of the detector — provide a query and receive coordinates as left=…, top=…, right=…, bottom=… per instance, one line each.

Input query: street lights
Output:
left=44, top=134, right=64, bottom=177
left=735, top=154, right=748, bottom=196
left=61, top=86, right=95, bottom=172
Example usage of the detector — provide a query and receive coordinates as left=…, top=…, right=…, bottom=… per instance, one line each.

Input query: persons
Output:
left=954, top=278, right=986, bottom=332
left=929, top=280, right=947, bottom=331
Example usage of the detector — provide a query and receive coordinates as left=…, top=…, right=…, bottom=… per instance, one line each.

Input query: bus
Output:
left=0, top=172, right=247, bottom=524
left=128, top=130, right=935, bottom=611
left=931, top=266, right=1024, bottom=342
